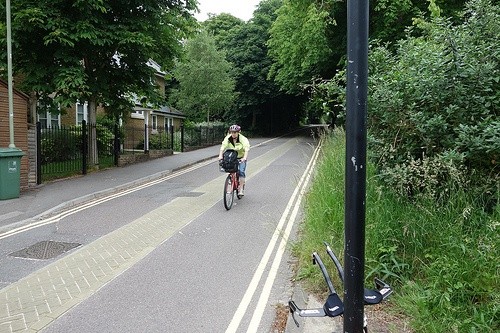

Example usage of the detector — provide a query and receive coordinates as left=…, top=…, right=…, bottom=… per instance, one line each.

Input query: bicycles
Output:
left=218, top=160, right=246, bottom=210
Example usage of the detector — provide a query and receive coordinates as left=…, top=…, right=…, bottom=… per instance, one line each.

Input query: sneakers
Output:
left=229, top=179, right=232, bottom=185
left=238, top=190, right=245, bottom=196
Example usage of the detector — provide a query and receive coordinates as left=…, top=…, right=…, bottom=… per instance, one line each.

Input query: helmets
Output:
left=230, top=125, right=241, bottom=132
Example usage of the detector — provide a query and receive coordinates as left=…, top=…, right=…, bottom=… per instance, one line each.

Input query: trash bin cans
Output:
left=0, top=146, right=26, bottom=202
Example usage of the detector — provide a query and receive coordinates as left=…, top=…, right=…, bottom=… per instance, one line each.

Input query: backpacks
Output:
left=223, top=149, right=238, bottom=171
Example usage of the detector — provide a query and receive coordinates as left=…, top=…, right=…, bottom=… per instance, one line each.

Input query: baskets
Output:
left=219, top=161, right=239, bottom=173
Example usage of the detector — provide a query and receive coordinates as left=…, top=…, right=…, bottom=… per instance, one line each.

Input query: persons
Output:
left=219, top=124, right=249, bottom=196
